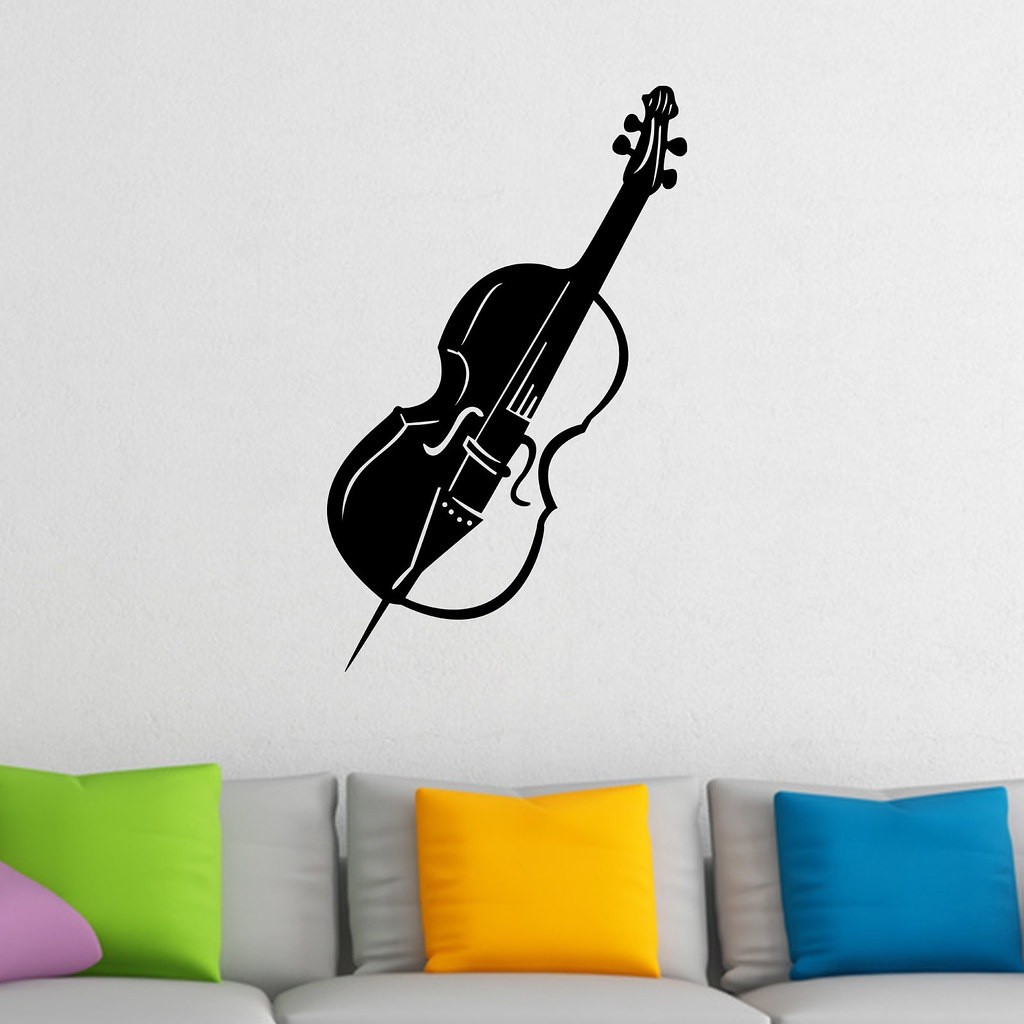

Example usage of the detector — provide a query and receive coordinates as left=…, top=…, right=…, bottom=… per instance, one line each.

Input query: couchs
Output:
left=0, top=764, right=1024, bottom=1024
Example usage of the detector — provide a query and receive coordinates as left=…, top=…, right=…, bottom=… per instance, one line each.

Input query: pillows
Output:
left=0, top=862, right=104, bottom=981
left=412, top=777, right=661, bottom=980
left=0, top=760, right=226, bottom=983
left=766, top=784, right=1024, bottom=977
